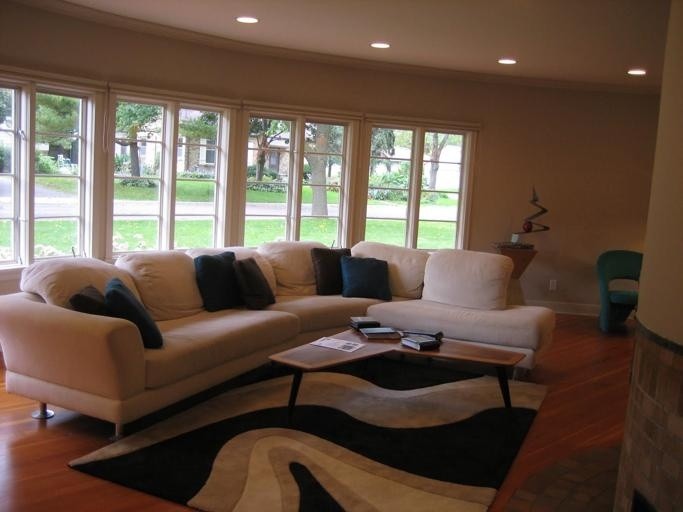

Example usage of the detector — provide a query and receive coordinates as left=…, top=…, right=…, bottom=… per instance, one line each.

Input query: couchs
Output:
left=1, top=241, right=555, bottom=439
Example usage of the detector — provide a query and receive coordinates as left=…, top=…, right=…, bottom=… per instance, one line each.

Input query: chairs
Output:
left=596, top=248, right=643, bottom=333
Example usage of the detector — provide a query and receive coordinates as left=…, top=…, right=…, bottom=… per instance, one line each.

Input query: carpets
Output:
left=67, top=355, right=549, bottom=511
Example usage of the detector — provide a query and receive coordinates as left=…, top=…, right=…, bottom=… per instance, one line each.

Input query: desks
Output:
left=267, top=325, right=527, bottom=414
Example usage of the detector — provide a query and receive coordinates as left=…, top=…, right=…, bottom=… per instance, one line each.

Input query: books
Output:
left=359, top=326, right=401, bottom=341
left=402, top=334, right=440, bottom=352
left=350, top=317, right=380, bottom=332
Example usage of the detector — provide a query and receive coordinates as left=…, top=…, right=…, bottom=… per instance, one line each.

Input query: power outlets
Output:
left=549, top=279, right=556, bottom=291
